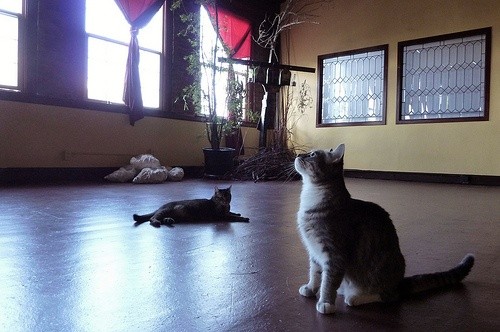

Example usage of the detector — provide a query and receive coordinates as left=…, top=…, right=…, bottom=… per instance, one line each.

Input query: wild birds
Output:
left=258, top=11, right=275, bottom=47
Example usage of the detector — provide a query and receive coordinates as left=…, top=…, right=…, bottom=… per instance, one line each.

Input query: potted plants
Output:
left=169, top=0, right=262, bottom=179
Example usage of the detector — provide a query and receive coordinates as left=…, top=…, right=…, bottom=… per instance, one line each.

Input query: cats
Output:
left=293, top=143, right=475, bottom=315
left=132, top=184, right=250, bottom=227
